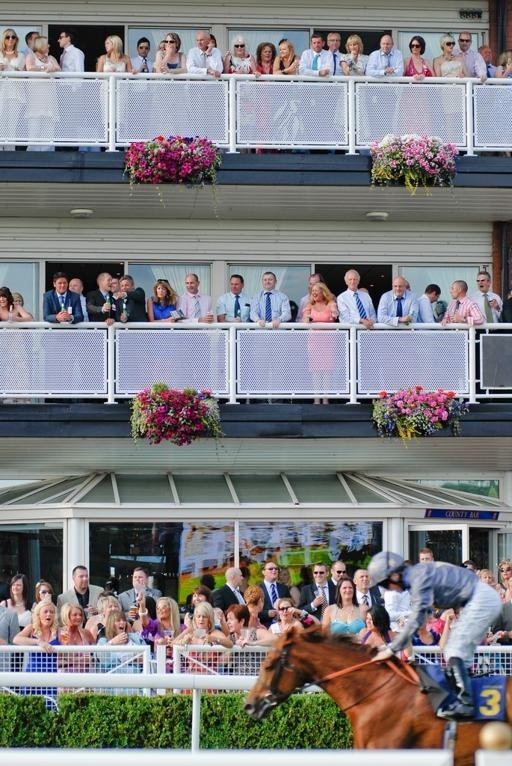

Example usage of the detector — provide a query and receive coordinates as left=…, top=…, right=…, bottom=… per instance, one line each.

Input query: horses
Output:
left=242, top=623, right=512, bottom=766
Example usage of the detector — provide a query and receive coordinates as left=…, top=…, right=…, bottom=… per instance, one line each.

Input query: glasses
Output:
left=269, top=568, right=278, bottom=570
left=314, top=572, right=325, bottom=574
left=446, top=42, right=455, bottom=46
left=235, top=45, right=245, bottom=48
left=410, top=45, right=420, bottom=48
left=6, top=35, right=15, bottom=38
left=140, top=47, right=149, bottom=49
left=41, top=589, right=52, bottom=593
left=337, top=571, right=345, bottom=573
left=460, top=39, right=471, bottom=42
left=500, top=567, right=511, bottom=572
left=165, top=41, right=176, bottom=43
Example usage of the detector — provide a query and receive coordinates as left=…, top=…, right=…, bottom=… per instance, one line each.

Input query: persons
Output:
left=1, top=548, right=511, bottom=688
left=1, top=268, right=512, bottom=400
left=1, top=29, right=512, bottom=155
left=364, top=550, right=503, bottom=718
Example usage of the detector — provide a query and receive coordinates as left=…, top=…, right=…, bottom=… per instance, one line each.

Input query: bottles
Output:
left=105, top=292, right=110, bottom=304
left=490, top=633, right=499, bottom=643
left=61, top=303, right=66, bottom=311
left=7, top=304, right=13, bottom=323
left=410, top=304, right=417, bottom=316
left=122, top=301, right=128, bottom=319
left=141, top=55, right=150, bottom=79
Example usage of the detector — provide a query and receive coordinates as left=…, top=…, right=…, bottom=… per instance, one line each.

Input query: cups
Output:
left=129, top=606, right=138, bottom=618
left=313, top=589, right=323, bottom=597
left=60, top=629, right=68, bottom=642
left=205, top=313, right=214, bottom=323
left=163, top=630, right=174, bottom=636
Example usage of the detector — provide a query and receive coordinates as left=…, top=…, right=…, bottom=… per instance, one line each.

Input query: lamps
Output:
left=68, top=208, right=97, bottom=219
left=363, top=211, right=389, bottom=221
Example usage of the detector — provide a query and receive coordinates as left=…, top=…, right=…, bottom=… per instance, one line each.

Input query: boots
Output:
left=437, top=656, right=475, bottom=717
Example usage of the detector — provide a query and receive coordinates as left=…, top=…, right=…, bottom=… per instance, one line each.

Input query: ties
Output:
left=271, top=584, right=277, bottom=604
left=312, top=54, right=320, bottom=70
left=333, top=54, right=336, bottom=75
left=362, top=595, right=368, bottom=606
left=235, top=296, right=241, bottom=318
left=487, top=66, right=490, bottom=76
left=265, top=292, right=272, bottom=321
left=396, top=298, right=402, bottom=316
left=455, top=299, right=460, bottom=312
left=320, top=587, right=328, bottom=616
left=483, top=294, right=493, bottom=323
left=353, top=293, right=367, bottom=319
left=59, top=296, right=64, bottom=306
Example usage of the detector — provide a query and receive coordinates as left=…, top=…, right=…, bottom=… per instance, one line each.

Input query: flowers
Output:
left=128, top=381, right=229, bottom=465
left=123, top=134, right=223, bottom=218
left=370, top=385, right=472, bottom=449
left=367, top=132, right=461, bottom=204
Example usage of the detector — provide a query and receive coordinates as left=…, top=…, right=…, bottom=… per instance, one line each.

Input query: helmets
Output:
left=367, top=551, right=404, bottom=586
